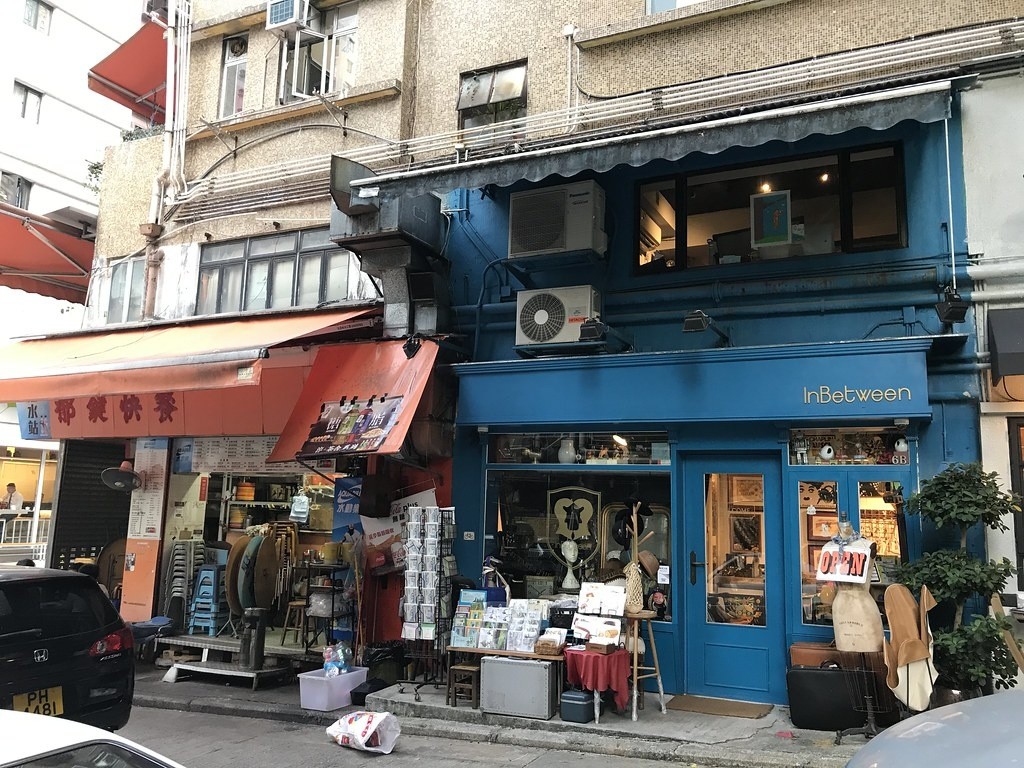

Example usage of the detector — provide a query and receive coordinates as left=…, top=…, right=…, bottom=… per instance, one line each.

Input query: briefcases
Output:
left=787, top=660, right=879, bottom=731
left=791, top=638, right=885, bottom=680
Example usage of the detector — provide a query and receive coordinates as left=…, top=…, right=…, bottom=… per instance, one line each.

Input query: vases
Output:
left=557, top=439, right=576, bottom=464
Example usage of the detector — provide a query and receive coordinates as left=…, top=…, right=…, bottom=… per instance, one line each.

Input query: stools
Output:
left=161, top=531, right=227, bottom=638
left=449, top=661, right=479, bottom=709
left=267, top=516, right=320, bottom=648
left=617, top=608, right=670, bottom=722
left=112, top=581, right=124, bottom=598
left=0, top=517, right=50, bottom=544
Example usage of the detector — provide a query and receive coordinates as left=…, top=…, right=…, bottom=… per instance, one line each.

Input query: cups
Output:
left=319, top=542, right=337, bottom=564
left=338, top=542, right=354, bottom=562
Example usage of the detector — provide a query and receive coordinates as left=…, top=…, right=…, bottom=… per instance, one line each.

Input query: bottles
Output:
left=347, top=403, right=373, bottom=445
left=331, top=404, right=360, bottom=445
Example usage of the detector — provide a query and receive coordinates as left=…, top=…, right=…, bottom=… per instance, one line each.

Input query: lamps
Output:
left=933, top=296, right=972, bottom=327
left=680, top=304, right=732, bottom=341
left=398, top=331, right=470, bottom=360
left=575, top=315, right=633, bottom=350
left=612, top=433, right=633, bottom=446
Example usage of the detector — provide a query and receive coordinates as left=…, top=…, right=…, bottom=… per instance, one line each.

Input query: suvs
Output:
left=0, top=564, right=135, bottom=733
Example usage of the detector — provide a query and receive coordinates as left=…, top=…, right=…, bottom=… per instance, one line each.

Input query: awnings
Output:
left=0, top=200, right=96, bottom=307
left=348, top=79, right=952, bottom=194
left=265, top=338, right=439, bottom=499
left=0, top=306, right=380, bottom=402
left=88, top=14, right=177, bottom=127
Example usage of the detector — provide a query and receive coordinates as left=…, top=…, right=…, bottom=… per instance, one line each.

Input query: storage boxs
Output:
left=479, top=653, right=556, bottom=722
left=560, top=689, right=604, bottom=723
left=295, top=665, right=372, bottom=715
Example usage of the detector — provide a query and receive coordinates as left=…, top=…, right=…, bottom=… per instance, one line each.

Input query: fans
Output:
left=102, top=460, right=146, bottom=492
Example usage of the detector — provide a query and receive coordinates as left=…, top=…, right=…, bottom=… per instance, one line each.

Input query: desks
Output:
left=215, top=534, right=251, bottom=638
left=442, top=643, right=563, bottom=714
left=253, top=536, right=279, bottom=636
left=236, top=533, right=264, bottom=638
left=97, top=534, right=126, bottom=587
left=565, top=643, right=633, bottom=725
left=0, top=508, right=29, bottom=516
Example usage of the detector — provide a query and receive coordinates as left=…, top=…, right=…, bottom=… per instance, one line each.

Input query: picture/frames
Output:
left=805, top=511, right=839, bottom=541
left=749, top=188, right=793, bottom=248
left=729, top=513, right=762, bottom=556
left=807, top=544, right=827, bottom=572
left=726, top=474, right=764, bottom=508
left=800, top=482, right=837, bottom=510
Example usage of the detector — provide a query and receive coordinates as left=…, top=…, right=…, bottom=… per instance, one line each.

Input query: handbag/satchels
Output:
left=326, top=711, right=401, bottom=754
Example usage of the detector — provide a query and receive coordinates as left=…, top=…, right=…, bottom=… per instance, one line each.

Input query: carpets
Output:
left=662, top=693, right=778, bottom=721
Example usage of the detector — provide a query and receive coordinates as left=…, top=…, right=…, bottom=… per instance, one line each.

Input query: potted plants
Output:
left=884, top=461, right=1024, bottom=703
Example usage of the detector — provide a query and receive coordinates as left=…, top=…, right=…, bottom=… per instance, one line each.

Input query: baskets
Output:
left=534, top=641, right=567, bottom=655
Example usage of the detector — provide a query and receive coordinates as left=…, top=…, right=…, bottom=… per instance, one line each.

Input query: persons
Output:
left=0, top=483, right=23, bottom=543
left=49, top=453, right=56, bottom=460
left=72, top=564, right=109, bottom=612
left=16, top=559, right=40, bottom=611
left=6, top=451, right=11, bottom=457
left=13, top=451, right=21, bottom=457
left=822, top=522, right=884, bottom=652
left=561, top=541, right=580, bottom=589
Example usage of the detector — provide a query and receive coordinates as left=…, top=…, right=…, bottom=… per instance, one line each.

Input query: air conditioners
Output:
left=512, top=285, right=602, bottom=343
left=264, top=0, right=319, bottom=41
left=506, top=178, right=607, bottom=258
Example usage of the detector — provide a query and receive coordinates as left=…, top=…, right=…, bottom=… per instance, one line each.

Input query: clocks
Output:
left=524, top=575, right=557, bottom=599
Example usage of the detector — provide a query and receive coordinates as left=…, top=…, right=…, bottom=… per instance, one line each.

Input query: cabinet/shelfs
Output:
left=305, top=562, right=353, bottom=652
left=223, top=473, right=333, bottom=536
left=289, top=566, right=331, bottom=600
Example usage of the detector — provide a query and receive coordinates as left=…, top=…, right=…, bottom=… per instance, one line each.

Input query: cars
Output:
left=0, top=709, right=187, bottom=768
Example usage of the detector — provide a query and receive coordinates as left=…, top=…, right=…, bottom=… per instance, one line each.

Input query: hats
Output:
left=638, top=549, right=660, bottom=580
